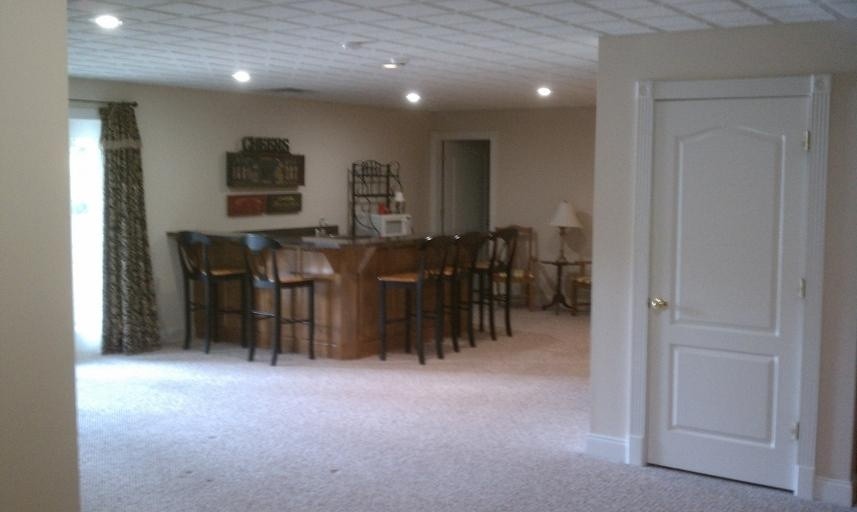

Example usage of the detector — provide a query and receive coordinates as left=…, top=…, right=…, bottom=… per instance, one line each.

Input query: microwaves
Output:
left=365, top=213, right=412, bottom=239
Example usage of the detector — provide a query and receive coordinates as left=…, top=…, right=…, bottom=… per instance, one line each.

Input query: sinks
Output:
left=302, top=235, right=354, bottom=244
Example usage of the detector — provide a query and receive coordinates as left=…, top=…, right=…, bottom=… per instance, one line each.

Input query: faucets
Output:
left=319, top=217, right=328, bottom=236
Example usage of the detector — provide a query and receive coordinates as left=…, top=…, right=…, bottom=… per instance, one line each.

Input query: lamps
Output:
left=549, top=198, right=584, bottom=262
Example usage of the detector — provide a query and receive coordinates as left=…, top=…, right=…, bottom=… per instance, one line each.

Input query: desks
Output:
left=537, top=256, right=591, bottom=317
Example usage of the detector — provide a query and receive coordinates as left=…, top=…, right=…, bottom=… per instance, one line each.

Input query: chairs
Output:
left=372, top=224, right=539, bottom=365
left=175, top=231, right=316, bottom=367
